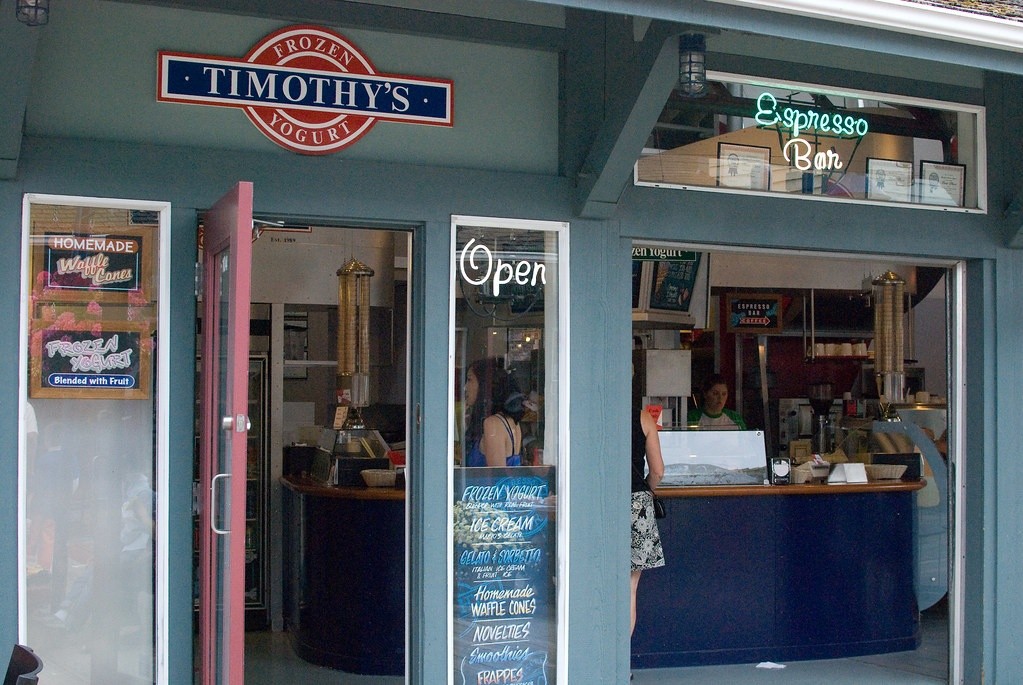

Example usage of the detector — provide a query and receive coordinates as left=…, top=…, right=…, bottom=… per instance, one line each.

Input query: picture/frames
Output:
left=31, top=319, right=149, bottom=400
left=724, top=294, right=782, bottom=334
left=39, top=222, right=152, bottom=306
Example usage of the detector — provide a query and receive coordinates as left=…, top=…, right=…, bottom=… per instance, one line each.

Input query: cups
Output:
left=843, top=392, right=851, bottom=401
left=905, top=392, right=946, bottom=405
left=806, top=343, right=867, bottom=357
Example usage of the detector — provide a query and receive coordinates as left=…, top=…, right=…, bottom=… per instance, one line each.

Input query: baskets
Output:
left=865, top=463, right=908, bottom=479
left=360, top=469, right=397, bottom=486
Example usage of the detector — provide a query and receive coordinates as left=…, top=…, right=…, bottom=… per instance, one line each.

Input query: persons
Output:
left=462, top=359, right=525, bottom=467
left=630, top=407, right=666, bottom=637
left=688, top=375, right=746, bottom=430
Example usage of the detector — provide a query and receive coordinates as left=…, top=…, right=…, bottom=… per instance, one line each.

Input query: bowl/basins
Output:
left=865, top=464, right=908, bottom=479
left=360, top=469, right=396, bottom=486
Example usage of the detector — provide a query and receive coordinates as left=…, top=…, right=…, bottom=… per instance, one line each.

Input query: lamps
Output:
left=679, top=33, right=708, bottom=98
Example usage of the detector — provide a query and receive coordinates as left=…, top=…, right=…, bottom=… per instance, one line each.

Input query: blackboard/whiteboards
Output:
left=32, top=220, right=152, bottom=304
left=453, top=466, right=558, bottom=685
left=30, top=319, right=151, bottom=400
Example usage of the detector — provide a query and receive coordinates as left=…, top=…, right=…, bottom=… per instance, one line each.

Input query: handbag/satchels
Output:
left=653, top=497, right=667, bottom=519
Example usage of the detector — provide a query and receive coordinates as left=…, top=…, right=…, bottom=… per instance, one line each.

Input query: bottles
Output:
left=828, top=416, right=873, bottom=454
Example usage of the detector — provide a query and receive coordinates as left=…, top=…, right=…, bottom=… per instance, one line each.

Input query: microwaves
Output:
left=860, top=364, right=925, bottom=404
